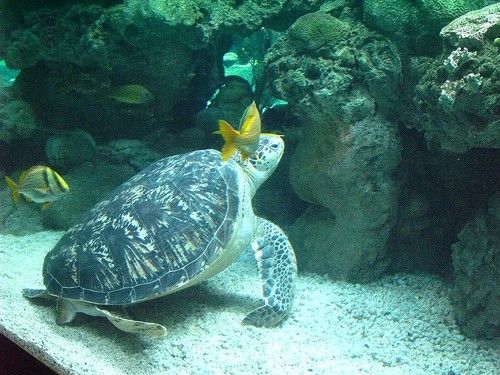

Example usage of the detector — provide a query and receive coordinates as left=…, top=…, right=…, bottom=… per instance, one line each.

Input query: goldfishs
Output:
left=212, top=100, right=285, bottom=163
left=4, top=166, right=69, bottom=209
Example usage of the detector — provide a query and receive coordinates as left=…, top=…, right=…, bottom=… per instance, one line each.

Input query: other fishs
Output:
left=105, top=84, right=154, bottom=104
